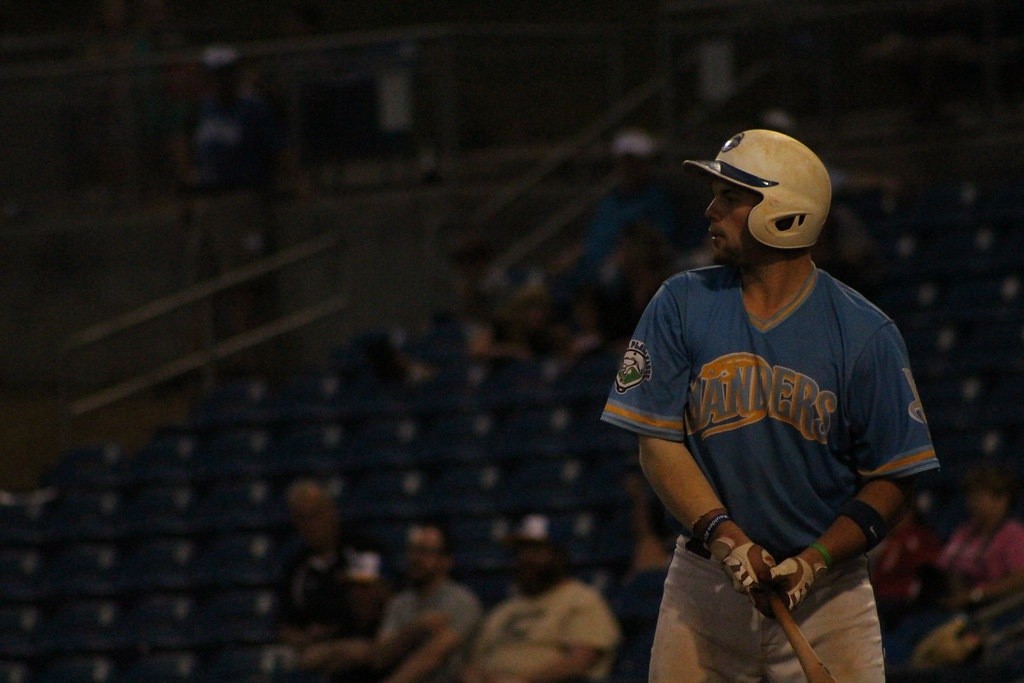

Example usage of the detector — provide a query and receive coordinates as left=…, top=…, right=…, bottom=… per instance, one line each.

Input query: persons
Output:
left=600, top=129, right=942, bottom=683
left=241, top=471, right=682, bottom=683
left=73, top=0, right=888, bottom=382
left=867, top=466, right=1024, bottom=633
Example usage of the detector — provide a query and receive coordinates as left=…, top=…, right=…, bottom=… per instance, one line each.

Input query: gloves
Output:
left=708, top=527, right=828, bottom=621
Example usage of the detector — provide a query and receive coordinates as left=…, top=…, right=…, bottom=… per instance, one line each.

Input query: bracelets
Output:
left=842, top=497, right=889, bottom=552
left=685, top=508, right=730, bottom=559
left=806, top=538, right=836, bottom=574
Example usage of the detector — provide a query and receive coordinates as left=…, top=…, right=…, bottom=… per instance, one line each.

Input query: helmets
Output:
left=682, top=128, right=831, bottom=250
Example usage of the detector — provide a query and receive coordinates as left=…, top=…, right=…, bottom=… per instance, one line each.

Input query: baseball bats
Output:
left=763, top=589, right=839, bottom=682
